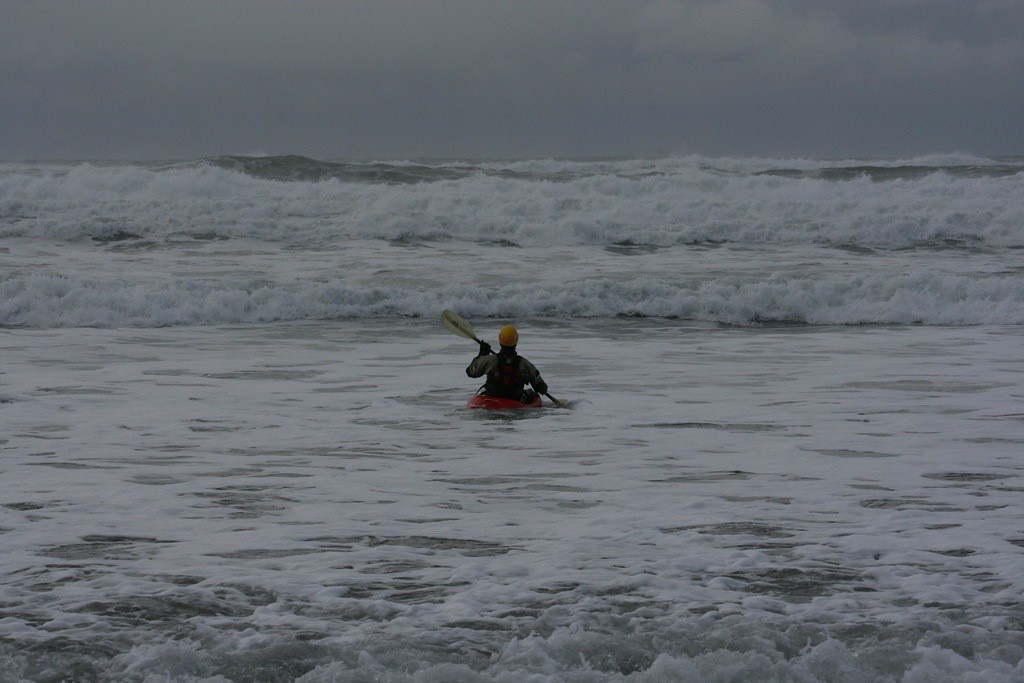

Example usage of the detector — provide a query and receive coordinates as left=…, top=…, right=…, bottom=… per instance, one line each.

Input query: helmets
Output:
left=499, top=326, right=519, bottom=346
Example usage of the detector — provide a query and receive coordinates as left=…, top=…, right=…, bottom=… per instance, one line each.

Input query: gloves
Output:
left=539, top=384, right=548, bottom=395
left=479, top=339, right=491, bottom=355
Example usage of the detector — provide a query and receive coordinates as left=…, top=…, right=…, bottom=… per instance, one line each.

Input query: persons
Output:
left=466, top=325, right=548, bottom=404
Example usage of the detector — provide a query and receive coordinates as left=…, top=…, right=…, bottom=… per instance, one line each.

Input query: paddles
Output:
left=440, top=309, right=568, bottom=408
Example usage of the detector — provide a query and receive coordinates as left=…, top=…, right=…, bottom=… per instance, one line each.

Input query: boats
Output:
left=469, top=391, right=542, bottom=410
